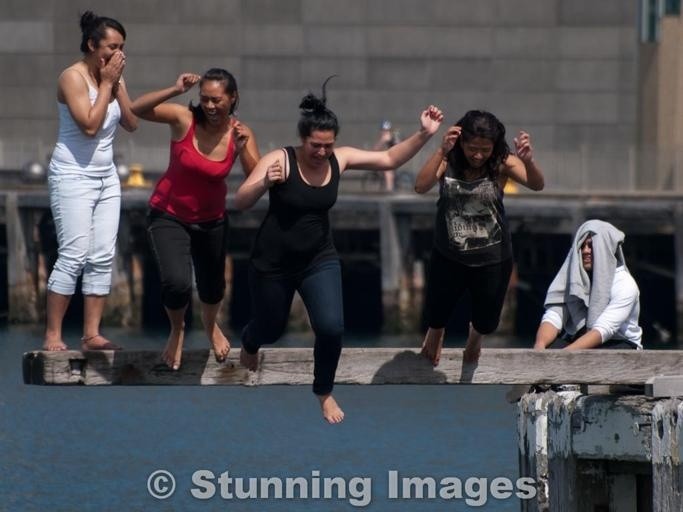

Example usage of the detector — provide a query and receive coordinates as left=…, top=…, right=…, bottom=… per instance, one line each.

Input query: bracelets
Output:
left=112, top=80, right=122, bottom=89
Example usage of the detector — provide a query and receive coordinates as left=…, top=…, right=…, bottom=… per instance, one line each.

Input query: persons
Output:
left=41, top=8, right=140, bottom=352
left=414, top=109, right=545, bottom=368
left=233, top=73, right=445, bottom=425
left=370, top=118, right=402, bottom=191
left=505, top=218, right=644, bottom=403
left=128, top=66, right=262, bottom=371
